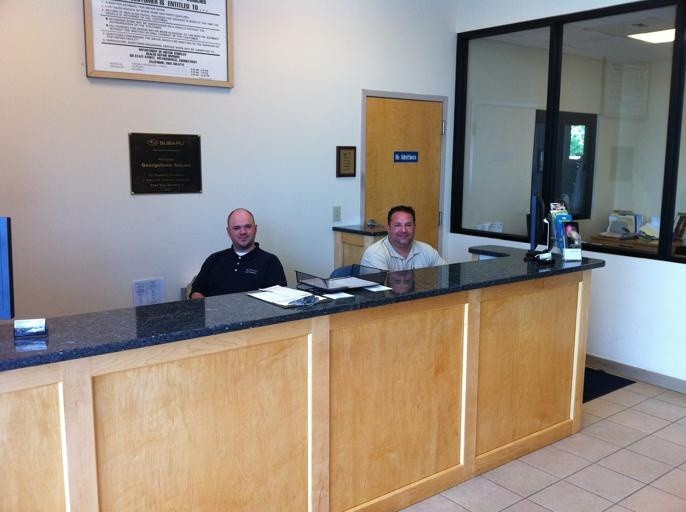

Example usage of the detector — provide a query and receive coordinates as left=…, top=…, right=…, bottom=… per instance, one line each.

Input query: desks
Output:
left=589, top=233, right=682, bottom=256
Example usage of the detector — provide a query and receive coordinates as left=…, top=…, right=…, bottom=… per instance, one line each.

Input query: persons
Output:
left=359, top=206, right=448, bottom=276
left=565, top=224, right=578, bottom=248
left=386, top=270, right=416, bottom=298
left=189, top=208, right=287, bottom=300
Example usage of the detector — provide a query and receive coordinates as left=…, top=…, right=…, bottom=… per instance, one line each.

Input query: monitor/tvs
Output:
left=526, top=191, right=556, bottom=259
left=0, top=217, right=15, bottom=321
